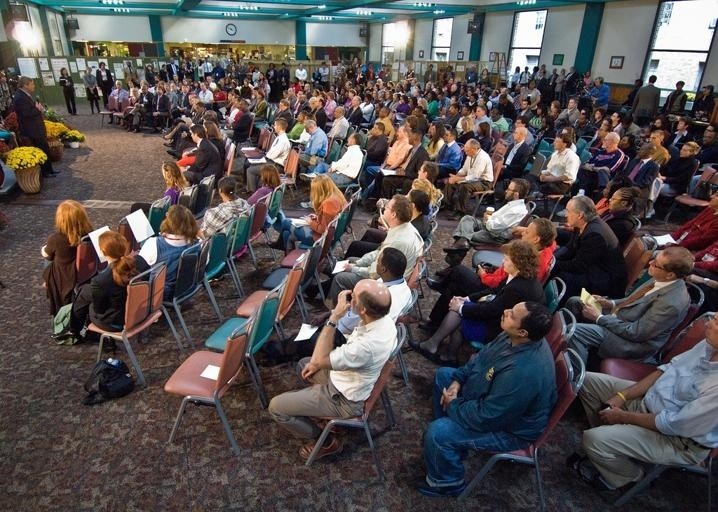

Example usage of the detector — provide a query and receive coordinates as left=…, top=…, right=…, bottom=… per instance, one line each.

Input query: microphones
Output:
left=34, top=94, right=42, bottom=105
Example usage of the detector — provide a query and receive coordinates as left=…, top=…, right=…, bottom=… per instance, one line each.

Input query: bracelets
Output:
left=324, top=319, right=336, bottom=327
left=616, top=391, right=627, bottom=403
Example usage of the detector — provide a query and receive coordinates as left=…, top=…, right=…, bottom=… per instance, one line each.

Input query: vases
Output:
left=47, top=136, right=80, bottom=162
left=15, top=166, right=43, bottom=193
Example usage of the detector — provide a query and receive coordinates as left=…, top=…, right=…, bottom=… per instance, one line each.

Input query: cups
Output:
left=485, top=206, right=495, bottom=215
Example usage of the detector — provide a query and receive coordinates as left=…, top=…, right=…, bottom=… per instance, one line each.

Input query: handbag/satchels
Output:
left=82, top=356, right=135, bottom=406
left=691, top=180, right=713, bottom=202
left=52, top=303, right=88, bottom=345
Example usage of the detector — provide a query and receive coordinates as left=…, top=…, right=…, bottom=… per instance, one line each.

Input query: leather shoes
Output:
left=46, top=174, right=56, bottom=177
left=408, top=338, right=441, bottom=363
left=419, top=319, right=438, bottom=332
left=426, top=278, right=443, bottom=295
left=163, top=135, right=171, bottom=140
left=435, top=354, right=457, bottom=368
left=443, top=242, right=470, bottom=253
left=52, top=170, right=60, bottom=173
left=436, top=265, right=456, bottom=277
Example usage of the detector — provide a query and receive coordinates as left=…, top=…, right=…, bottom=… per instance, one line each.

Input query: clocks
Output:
left=225, top=23, right=237, bottom=36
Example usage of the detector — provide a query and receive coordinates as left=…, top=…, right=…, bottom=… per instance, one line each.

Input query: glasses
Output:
left=654, top=254, right=665, bottom=272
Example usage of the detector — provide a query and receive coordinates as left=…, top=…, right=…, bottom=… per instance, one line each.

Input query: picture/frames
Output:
left=552, top=53, right=564, bottom=65
left=419, top=50, right=424, bottom=58
left=457, top=51, right=464, bottom=60
left=609, top=56, right=624, bottom=69
left=489, top=52, right=497, bottom=62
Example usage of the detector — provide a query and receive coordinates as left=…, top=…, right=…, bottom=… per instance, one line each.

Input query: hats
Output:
left=703, top=85, right=713, bottom=88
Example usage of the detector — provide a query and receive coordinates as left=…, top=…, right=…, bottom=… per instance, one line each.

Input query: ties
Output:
left=611, top=282, right=655, bottom=314
left=628, top=159, right=643, bottom=180
left=438, top=144, right=448, bottom=160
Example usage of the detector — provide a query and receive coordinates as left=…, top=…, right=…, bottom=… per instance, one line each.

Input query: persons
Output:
left=13, top=77, right=61, bottom=178
left=416, top=301, right=558, bottom=498
left=577, top=311, right=718, bottom=492
left=267, top=279, right=398, bottom=461
left=40, top=50, right=718, bottom=372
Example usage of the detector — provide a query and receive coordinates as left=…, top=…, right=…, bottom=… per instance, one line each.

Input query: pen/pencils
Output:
left=595, top=296, right=608, bottom=301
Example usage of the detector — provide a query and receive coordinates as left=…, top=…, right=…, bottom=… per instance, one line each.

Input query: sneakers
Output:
left=299, top=436, right=343, bottom=460
left=267, top=238, right=286, bottom=251
left=645, top=209, right=655, bottom=219
left=299, top=172, right=317, bottom=182
left=167, top=150, right=180, bottom=158
left=300, top=201, right=314, bottom=208
left=598, top=467, right=644, bottom=489
left=164, top=142, right=173, bottom=147
left=317, top=419, right=348, bottom=435
left=449, top=211, right=464, bottom=220
left=419, top=483, right=466, bottom=498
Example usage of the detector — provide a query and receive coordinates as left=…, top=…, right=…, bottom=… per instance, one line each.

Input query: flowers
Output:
left=43, top=107, right=87, bottom=143
left=6, top=145, right=52, bottom=168
